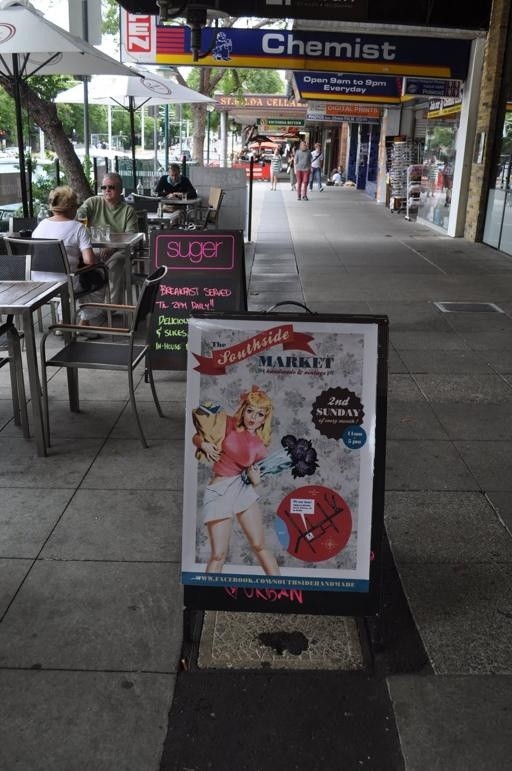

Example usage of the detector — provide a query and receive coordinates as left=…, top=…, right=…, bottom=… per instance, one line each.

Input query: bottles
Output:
left=37, top=205, right=49, bottom=226
left=136, top=180, right=143, bottom=196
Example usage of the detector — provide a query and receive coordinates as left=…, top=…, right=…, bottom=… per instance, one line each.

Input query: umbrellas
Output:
left=0, top=0, right=144, bottom=235
left=250, top=140, right=278, bottom=151
left=47, top=59, right=219, bottom=201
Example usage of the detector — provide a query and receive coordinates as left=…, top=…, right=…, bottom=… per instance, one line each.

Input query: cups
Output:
left=152, top=192, right=187, bottom=201
left=92, top=225, right=112, bottom=243
left=77, top=213, right=87, bottom=228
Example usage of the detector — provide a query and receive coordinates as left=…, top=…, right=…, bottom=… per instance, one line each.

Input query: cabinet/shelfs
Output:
left=405, top=164, right=424, bottom=221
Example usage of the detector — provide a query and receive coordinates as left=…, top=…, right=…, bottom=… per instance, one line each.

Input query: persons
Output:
left=338, top=165, right=346, bottom=185
left=238, top=149, right=271, bottom=166
left=261, top=144, right=283, bottom=191
left=309, top=142, right=324, bottom=192
left=190, top=390, right=282, bottom=577
left=26, top=183, right=108, bottom=339
left=154, top=163, right=196, bottom=229
left=293, top=140, right=313, bottom=201
left=288, top=146, right=298, bottom=192
left=76, top=172, right=137, bottom=318
left=328, top=168, right=342, bottom=186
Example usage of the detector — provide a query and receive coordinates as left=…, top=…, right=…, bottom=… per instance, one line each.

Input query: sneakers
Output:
left=297, top=195, right=308, bottom=200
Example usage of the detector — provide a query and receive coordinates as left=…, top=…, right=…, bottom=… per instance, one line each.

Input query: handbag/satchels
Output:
left=287, top=166, right=292, bottom=174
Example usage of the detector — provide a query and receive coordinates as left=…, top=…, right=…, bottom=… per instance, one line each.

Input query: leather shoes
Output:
left=76, top=326, right=101, bottom=339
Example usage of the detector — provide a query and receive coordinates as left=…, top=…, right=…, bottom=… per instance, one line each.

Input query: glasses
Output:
left=100, top=185, right=117, bottom=190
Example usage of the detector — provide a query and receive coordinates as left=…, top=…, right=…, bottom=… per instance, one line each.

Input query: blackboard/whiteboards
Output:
left=145, top=229, right=243, bottom=372
